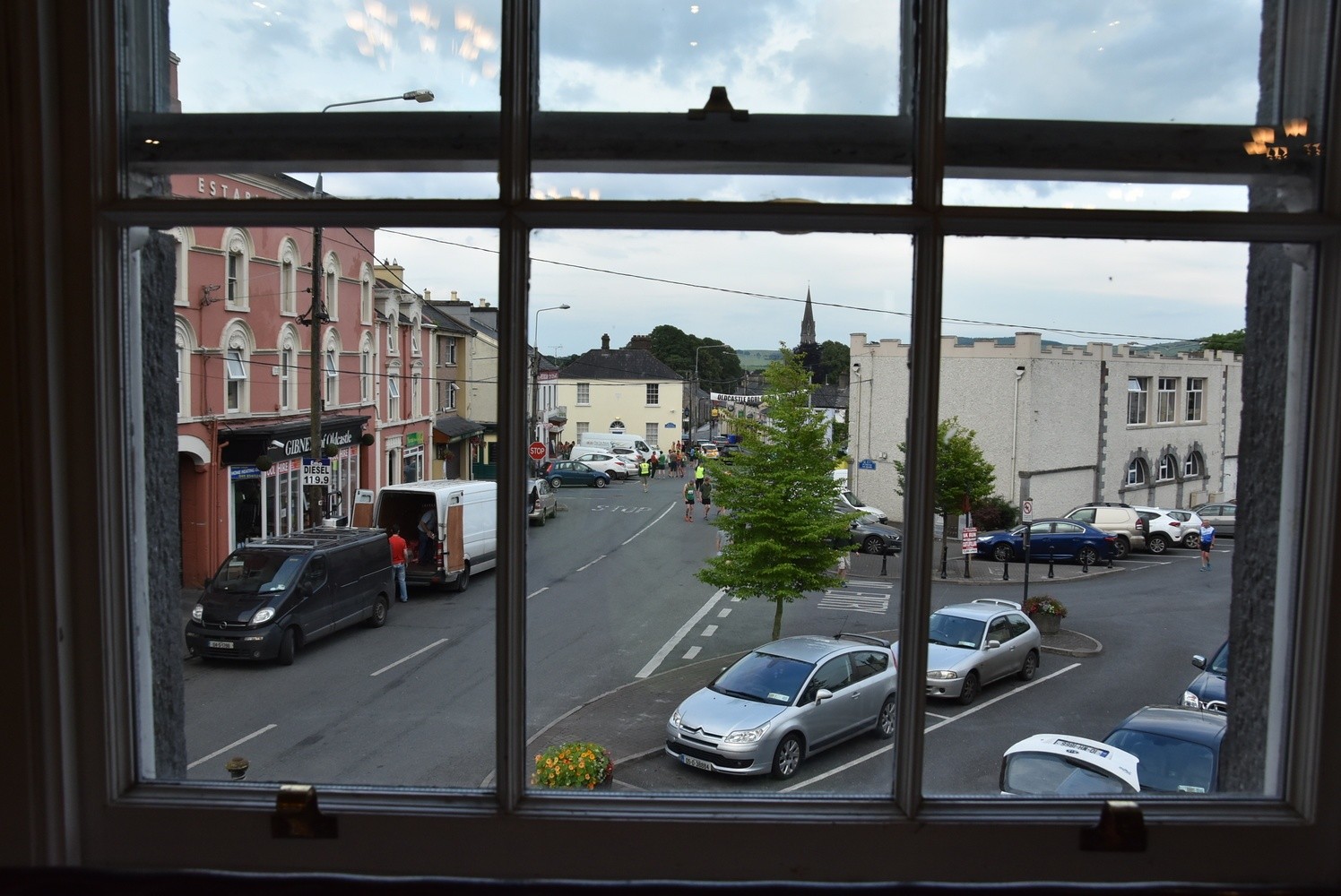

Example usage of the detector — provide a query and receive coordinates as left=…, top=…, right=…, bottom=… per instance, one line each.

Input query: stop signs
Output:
left=528, top=441, right=547, bottom=460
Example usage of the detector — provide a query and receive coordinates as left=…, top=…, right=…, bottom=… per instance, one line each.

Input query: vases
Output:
left=1029, top=612, right=1061, bottom=634
left=542, top=772, right=615, bottom=791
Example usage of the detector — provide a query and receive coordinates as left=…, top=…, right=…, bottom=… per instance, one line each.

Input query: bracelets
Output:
left=1211, top=544, right=1214, bottom=546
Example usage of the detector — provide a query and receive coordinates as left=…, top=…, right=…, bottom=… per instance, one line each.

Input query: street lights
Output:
left=695, top=344, right=732, bottom=442
left=529, top=304, right=571, bottom=478
left=721, top=351, right=747, bottom=421
left=310, top=89, right=438, bottom=527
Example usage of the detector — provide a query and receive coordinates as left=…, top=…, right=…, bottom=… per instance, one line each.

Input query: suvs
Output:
left=527, top=477, right=557, bottom=528
left=1130, top=505, right=1183, bottom=555
left=569, top=446, right=641, bottom=478
left=1032, top=502, right=1146, bottom=560
left=569, top=453, right=627, bottom=481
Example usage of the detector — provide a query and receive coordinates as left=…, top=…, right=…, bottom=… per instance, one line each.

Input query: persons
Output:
left=638, top=440, right=728, bottom=555
left=388, top=524, right=409, bottom=603
left=560, top=440, right=575, bottom=461
left=1197, top=519, right=1216, bottom=572
left=416, top=507, right=437, bottom=570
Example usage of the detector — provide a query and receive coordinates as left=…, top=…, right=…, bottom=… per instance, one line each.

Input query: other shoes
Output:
left=685, top=516, right=688, bottom=521
left=1207, top=563, right=1211, bottom=570
left=689, top=518, right=693, bottom=522
left=704, top=516, right=708, bottom=521
left=1200, top=567, right=1205, bottom=571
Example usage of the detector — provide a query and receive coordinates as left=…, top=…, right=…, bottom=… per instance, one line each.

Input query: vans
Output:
left=184, top=525, right=394, bottom=667
left=349, top=478, right=497, bottom=593
left=580, top=432, right=663, bottom=469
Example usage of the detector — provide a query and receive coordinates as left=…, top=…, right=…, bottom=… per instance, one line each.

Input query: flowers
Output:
left=530, top=743, right=612, bottom=791
left=1021, top=595, right=1068, bottom=619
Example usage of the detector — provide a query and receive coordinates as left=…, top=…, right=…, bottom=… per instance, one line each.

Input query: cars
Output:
left=889, top=598, right=1044, bottom=705
left=970, top=518, right=1119, bottom=566
left=1189, top=499, right=1236, bottom=539
left=537, top=460, right=611, bottom=489
left=1165, top=510, right=1204, bottom=550
left=810, top=485, right=902, bottom=555
left=1061, top=701, right=1230, bottom=790
left=662, top=630, right=900, bottom=780
left=697, top=436, right=743, bottom=465
left=1175, top=640, right=1230, bottom=711
